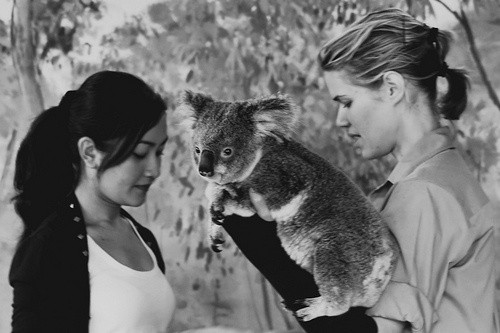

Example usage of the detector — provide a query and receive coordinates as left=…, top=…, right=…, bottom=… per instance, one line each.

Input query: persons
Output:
left=10, top=70, right=176, bottom=333
left=212, top=8, right=500, bottom=333
